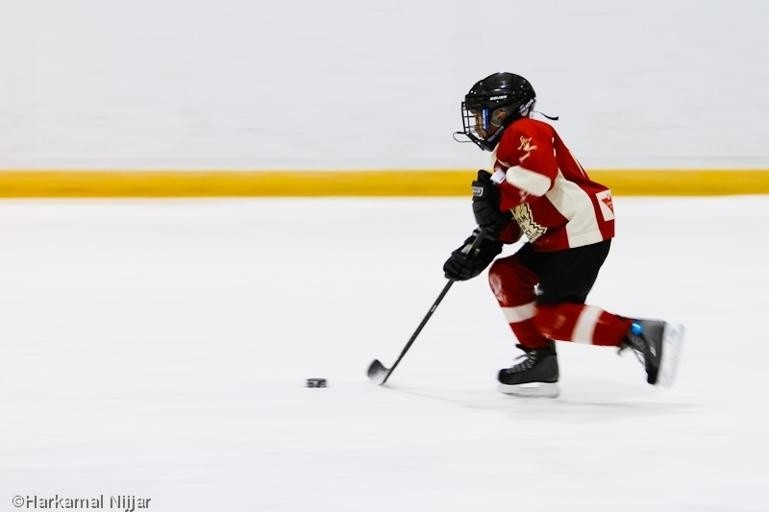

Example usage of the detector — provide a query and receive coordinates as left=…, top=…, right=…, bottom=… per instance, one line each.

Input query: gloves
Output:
left=472, top=171, right=523, bottom=243
left=443, top=224, right=501, bottom=281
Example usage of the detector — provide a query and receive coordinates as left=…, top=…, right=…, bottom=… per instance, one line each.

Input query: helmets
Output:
left=453, top=72, right=536, bottom=152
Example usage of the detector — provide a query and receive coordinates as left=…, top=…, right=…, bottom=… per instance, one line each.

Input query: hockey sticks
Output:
left=368, top=230, right=481, bottom=385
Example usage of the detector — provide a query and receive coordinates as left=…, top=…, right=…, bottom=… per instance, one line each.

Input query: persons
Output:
left=443, top=70, right=667, bottom=385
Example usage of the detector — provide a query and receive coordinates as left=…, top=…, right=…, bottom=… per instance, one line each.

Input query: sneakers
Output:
left=499, top=339, right=558, bottom=384
left=614, top=314, right=665, bottom=384
